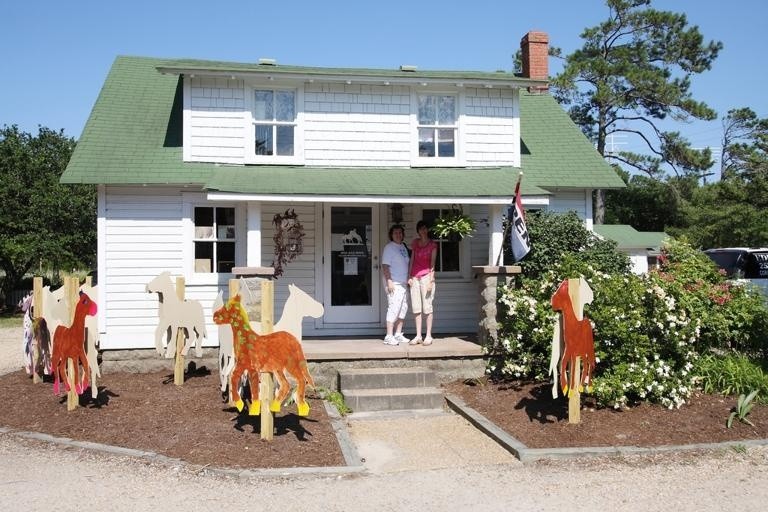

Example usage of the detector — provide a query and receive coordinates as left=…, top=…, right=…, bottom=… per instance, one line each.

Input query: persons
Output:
left=407, top=221, right=437, bottom=345
left=379, top=224, right=410, bottom=345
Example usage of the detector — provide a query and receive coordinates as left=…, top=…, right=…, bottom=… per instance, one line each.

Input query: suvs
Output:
left=700, top=245, right=768, bottom=305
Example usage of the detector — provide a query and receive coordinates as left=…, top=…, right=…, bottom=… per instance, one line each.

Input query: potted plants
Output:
left=431, top=204, right=477, bottom=243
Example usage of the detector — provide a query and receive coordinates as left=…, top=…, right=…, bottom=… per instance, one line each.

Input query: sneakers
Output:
left=423, top=336, right=432, bottom=345
left=395, top=333, right=410, bottom=342
left=409, top=336, right=422, bottom=344
left=384, top=335, right=398, bottom=345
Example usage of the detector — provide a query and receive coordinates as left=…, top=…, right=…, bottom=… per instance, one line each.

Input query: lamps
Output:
left=389, top=203, right=406, bottom=226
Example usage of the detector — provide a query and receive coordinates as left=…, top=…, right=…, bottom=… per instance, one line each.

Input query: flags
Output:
left=507, top=183, right=529, bottom=263
